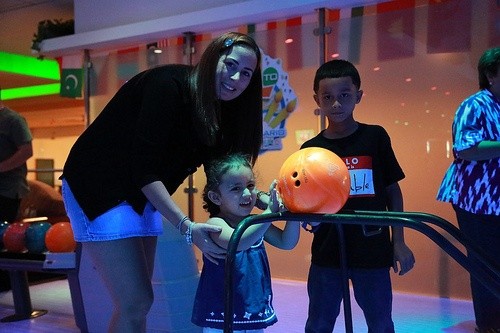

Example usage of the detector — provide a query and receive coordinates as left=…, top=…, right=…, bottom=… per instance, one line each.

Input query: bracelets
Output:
left=176, top=216, right=191, bottom=234
left=184, top=222, right=197, bottom=246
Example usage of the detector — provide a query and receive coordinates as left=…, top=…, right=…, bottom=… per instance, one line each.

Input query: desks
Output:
left=0, top=252, right=88, bottom=333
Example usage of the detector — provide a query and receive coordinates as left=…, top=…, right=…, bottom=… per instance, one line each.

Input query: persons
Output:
left=301, top=60, right=415, bottom=332
left=436, top=48, right=499, bottom=333
left=0, top=104, right=33, bottom=223
left=191, top=155, right=300, bottom=332
left=61, top=31, right=262, bottom=333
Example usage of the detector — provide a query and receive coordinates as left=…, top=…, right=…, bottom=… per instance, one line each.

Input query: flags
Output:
left=427, top=0, right=472, bottom=54
left=62, top=55, right=82, bottom=97
left=89, top=51, right=110, bottom=96
left=487, top=0, right=499, bottom=49
left=327, top=6, right=364, bottom=64
left=177, top=34, right=203, bottom=65
left=211, top=28, right=238, bottom=39
left=247, top=22, right=276, bottom=59
left=117, top=47, right=139, bottom=92
left=286, top=14, right=318, bottom=71
left=146, top=39, right=170, bottom=67
left=377, top=0, right=415, bottom=61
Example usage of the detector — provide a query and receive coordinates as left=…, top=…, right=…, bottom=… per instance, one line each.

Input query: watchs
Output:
left=256, top=190, right=270, bottom=209
